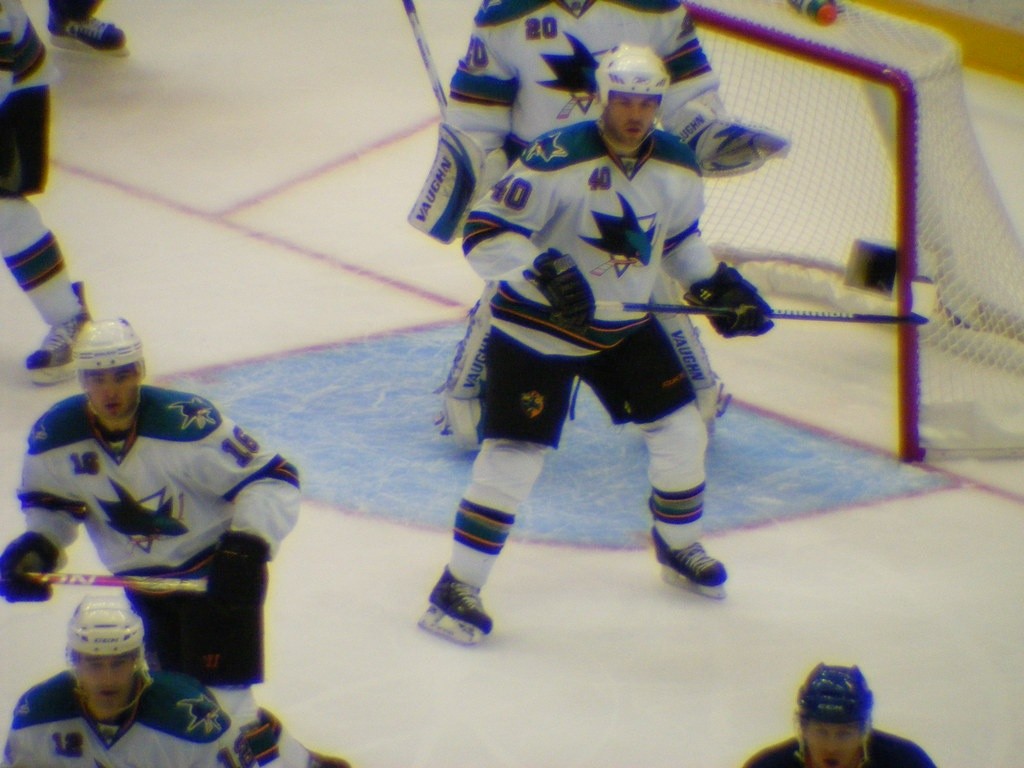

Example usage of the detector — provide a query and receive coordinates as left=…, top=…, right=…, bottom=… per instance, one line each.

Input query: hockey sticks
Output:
left=399, top=0, right=449, bottom=116
left=594, top=271, right=940, bottom=328
left=23, top=568, right=208, bottom=595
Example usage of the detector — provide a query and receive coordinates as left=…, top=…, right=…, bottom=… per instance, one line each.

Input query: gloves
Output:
left=536, top=252, right=595, bottom=332
left=689, top=264, right=773, bottom=338
left=0, top=532, right=65, bottom=602
left=208, top=528, right=271, bottom=620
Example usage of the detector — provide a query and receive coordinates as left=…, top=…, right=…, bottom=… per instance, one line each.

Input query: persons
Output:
left=407, top=0, right=790, bottom=454
left=0, top=319, right=350, bottom=768
left=741, top=661, right=937, bottom=768
left=0, top=0, right=96, bottom=370
left=0, top=590, right=261, bottom=768
left=48, top=0, right=126, bottom=50
left=429, top=42, right=774, bottom=635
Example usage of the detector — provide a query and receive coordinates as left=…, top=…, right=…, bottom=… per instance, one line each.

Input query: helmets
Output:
left=797, top=662, right=874, bottom=723
left=71, top=319, right=146, bottom=384
left=595, top=45, right=670, bottom=108
left=68, top=598, right=144, bottom=667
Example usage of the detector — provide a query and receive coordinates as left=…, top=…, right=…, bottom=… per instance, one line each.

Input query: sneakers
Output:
left=25, top=280, right=94, bottom=384
left=416, top=564, right=493, bottom=643
left=47, top=8, right=129, bottom=58
left=651, top=525, right=727, bottom=599
